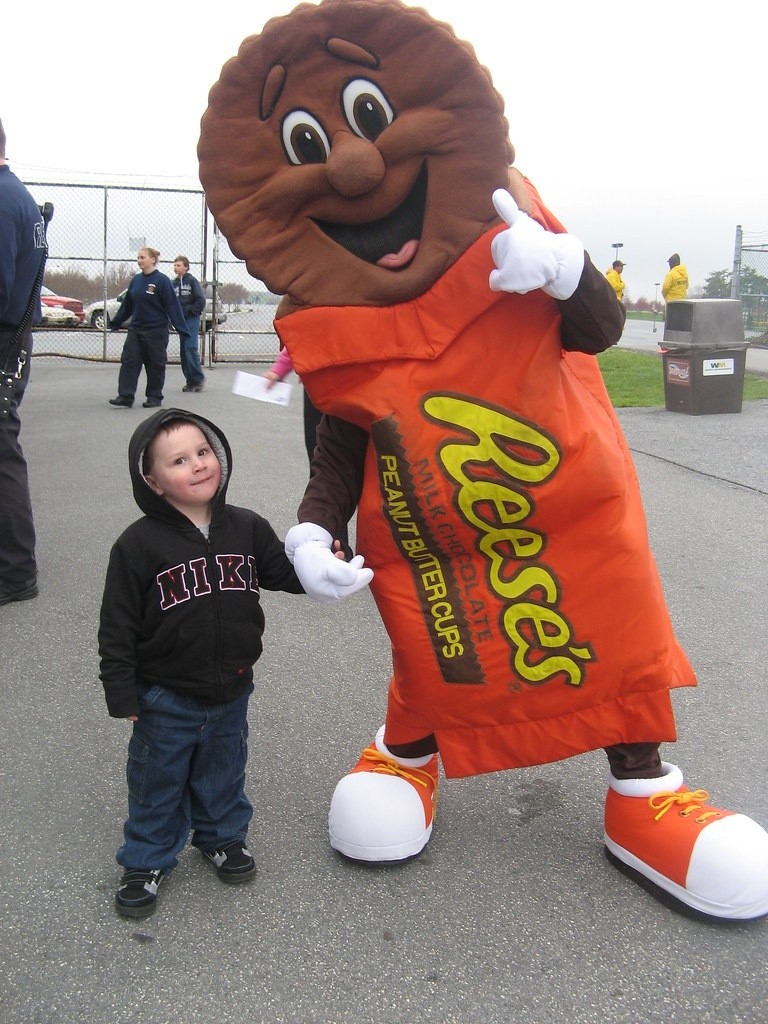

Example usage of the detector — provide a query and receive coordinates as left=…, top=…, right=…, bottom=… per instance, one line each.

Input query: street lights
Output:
left=611, top=242, right=623, bottom=263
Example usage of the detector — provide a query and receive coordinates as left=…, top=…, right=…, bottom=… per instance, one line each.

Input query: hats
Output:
left=613, top=261, right=625, bottom=267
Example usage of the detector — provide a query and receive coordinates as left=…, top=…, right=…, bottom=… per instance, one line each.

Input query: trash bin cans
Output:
left=659, top=299, right=751, bottom=413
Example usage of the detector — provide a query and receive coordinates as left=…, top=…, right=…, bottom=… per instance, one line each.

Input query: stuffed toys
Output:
left=196, top=0, right=768, bottom=922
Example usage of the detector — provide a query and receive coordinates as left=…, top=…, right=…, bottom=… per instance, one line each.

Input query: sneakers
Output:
left=204, top=844, right=257, bottom=883
left=114, top=863, right=160, bottom=922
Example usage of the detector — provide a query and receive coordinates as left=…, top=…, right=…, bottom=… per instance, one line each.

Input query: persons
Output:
left=98, top=407, right=348, bottom=920
left=605, top=260, right=626, bottom=345
left=109, top=247, right=193, bottom=408
left=0, top=119, right=49, bottom=605
left=171, top=255, right=206, bottom=392
left=263, top=346, right=354, bottom=563
left=661, top=253, right=688, bottom=303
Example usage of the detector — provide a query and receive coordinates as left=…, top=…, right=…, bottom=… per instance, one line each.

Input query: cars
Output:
left=41, top=286, right=85, bottom=322
left=38, top=302, right=79, bottom=328
left=84, top=288, right=228, bottom=334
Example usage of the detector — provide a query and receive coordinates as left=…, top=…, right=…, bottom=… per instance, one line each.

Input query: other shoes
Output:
left=0, top=577, right=38, bottom=605
left=183, top=377, right=205, bottom=392
left=144, top=397, right=163, bottom=408
left=109, top=395, right=133, bottom=407
